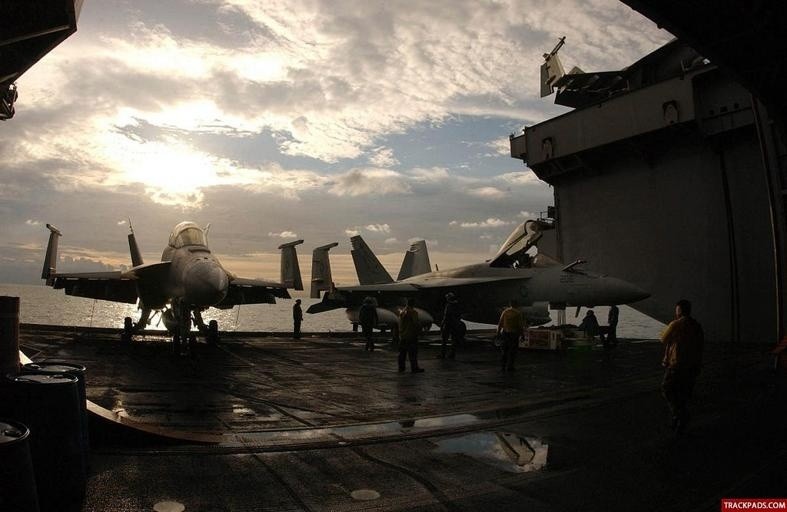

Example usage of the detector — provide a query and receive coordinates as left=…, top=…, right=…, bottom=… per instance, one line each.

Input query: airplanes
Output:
left=307, top=218, right=651, bottom=344
left=540, top=35, right=699, bottom=106
left=42, top=222, right=305, bottom=349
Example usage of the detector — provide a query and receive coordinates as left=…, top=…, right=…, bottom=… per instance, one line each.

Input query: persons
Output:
left=763, top=330, right=786, bottom=373
left=432, top=292, right=461, bottom=360
left=395, top=297, right=425, bottom=375
left=583, top=310, right=606, bottom=346
left=358, top=298, right=380, bottom=353
left=495, top=297, right=524, bottom=372
left=656, top=294, right=707, bottom=436
left=291, top=298, right=304, bottom=339
left=606, top=304, right=619, bottom=346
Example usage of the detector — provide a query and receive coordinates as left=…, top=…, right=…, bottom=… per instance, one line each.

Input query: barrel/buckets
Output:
left=0, top=361, right=86, bottom=512
